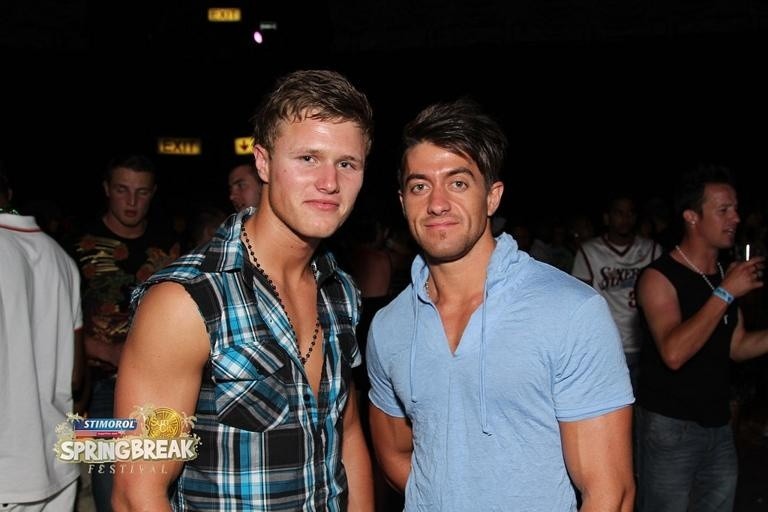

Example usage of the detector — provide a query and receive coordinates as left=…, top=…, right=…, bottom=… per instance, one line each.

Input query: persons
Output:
left=0, top=144, right=768, bottom=510
left=109, top=68, right=378, bottom=511
left=365, top=100, right=640, bottom=511
